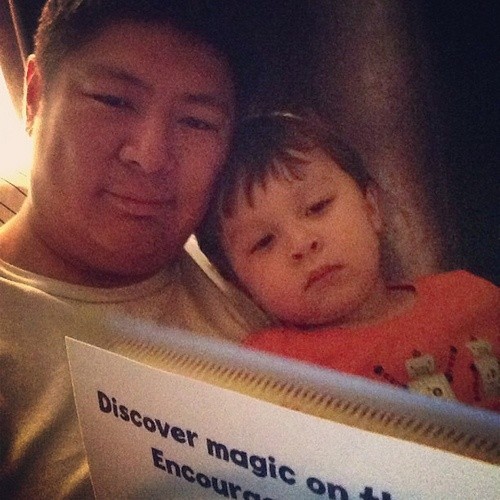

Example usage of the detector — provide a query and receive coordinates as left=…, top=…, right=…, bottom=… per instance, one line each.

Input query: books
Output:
left=64, top=310, right=498, bottom=498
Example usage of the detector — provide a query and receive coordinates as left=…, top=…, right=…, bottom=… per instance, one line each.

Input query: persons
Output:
left=0, top=0, right=269, bottom=499
left=194, top=105, right=499, bottom=500
